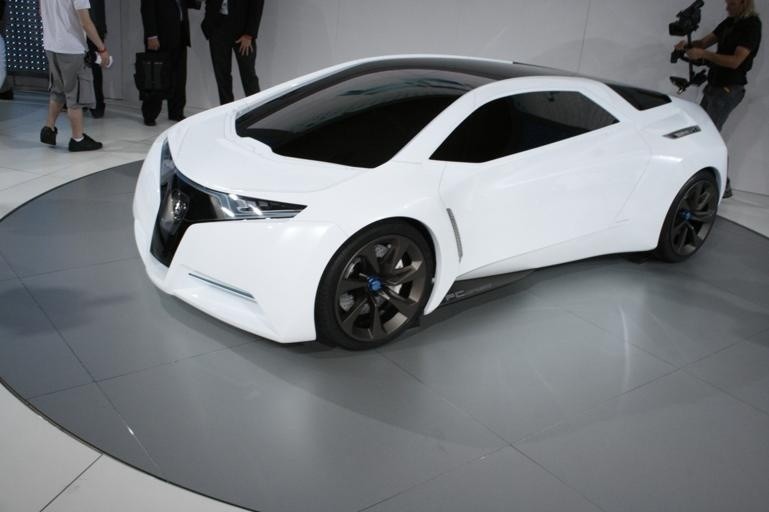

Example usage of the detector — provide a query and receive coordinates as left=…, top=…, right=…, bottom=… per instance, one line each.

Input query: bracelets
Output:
left=97, top=45, right=107, bottom=53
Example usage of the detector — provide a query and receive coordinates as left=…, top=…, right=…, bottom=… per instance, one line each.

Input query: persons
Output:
left=60, top=0, right=108, bottom=117
left=39, top=0, right=111, bottom=152
left=140, top=0, right=203, bottom=126
left=674, top=1, right=762, bottom=198
left=204, top=0, right=265, bottom=105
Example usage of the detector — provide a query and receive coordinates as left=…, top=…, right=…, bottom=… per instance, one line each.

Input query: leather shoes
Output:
left=168, top=114, right=186, bottom=122
left=144, top=117, right=155, bottom=126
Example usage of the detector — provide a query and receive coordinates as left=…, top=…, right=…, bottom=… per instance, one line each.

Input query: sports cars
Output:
left=133, top=53, right=731, bottom=351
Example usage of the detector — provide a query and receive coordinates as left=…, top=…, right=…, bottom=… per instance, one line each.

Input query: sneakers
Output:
left=40, top=125, right=58, bottom=145
left=68, top=132, right=102, bottom=152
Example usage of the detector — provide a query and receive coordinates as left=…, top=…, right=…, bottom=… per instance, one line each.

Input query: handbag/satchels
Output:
left=134, top=50, right=172, bottom=93
